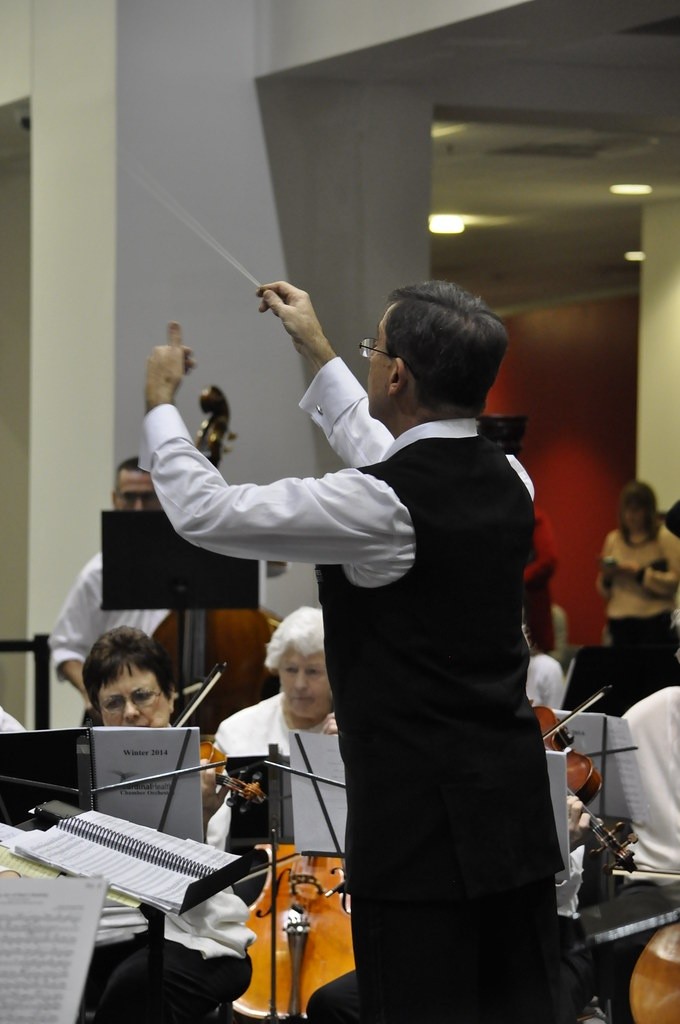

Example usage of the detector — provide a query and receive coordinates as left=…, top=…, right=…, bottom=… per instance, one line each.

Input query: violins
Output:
left=194, top=741, right=267, bottom=806
left=530, top=700, right=644, bottom=877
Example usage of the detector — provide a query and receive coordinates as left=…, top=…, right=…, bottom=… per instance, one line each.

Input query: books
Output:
left=10, top=811, right=242, bottom=914
left=0, top=726, right=204, bottom=844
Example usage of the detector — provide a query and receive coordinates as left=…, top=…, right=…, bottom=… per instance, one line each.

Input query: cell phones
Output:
left=603, top=556, right=617, bottom=568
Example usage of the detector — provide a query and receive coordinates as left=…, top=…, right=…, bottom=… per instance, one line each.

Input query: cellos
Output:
left=149, top=382, right=284, bottom=728
left=227, top=840, right=359, bottom=1020
left=624, top=923, right=680, bottom=1024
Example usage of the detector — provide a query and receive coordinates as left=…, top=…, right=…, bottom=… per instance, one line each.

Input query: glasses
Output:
left=99, top=688, right=164, bottom=714
left=357, top=337, right=398, bottom=360
left=116, top=490, right=157, bottom=502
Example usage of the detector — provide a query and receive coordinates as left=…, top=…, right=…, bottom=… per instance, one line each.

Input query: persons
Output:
left=48, top=455, right=229, bottom=842
left=138, top=280, right=603, bottom=1024
left=596, top=480, right=680, bottom=1024
